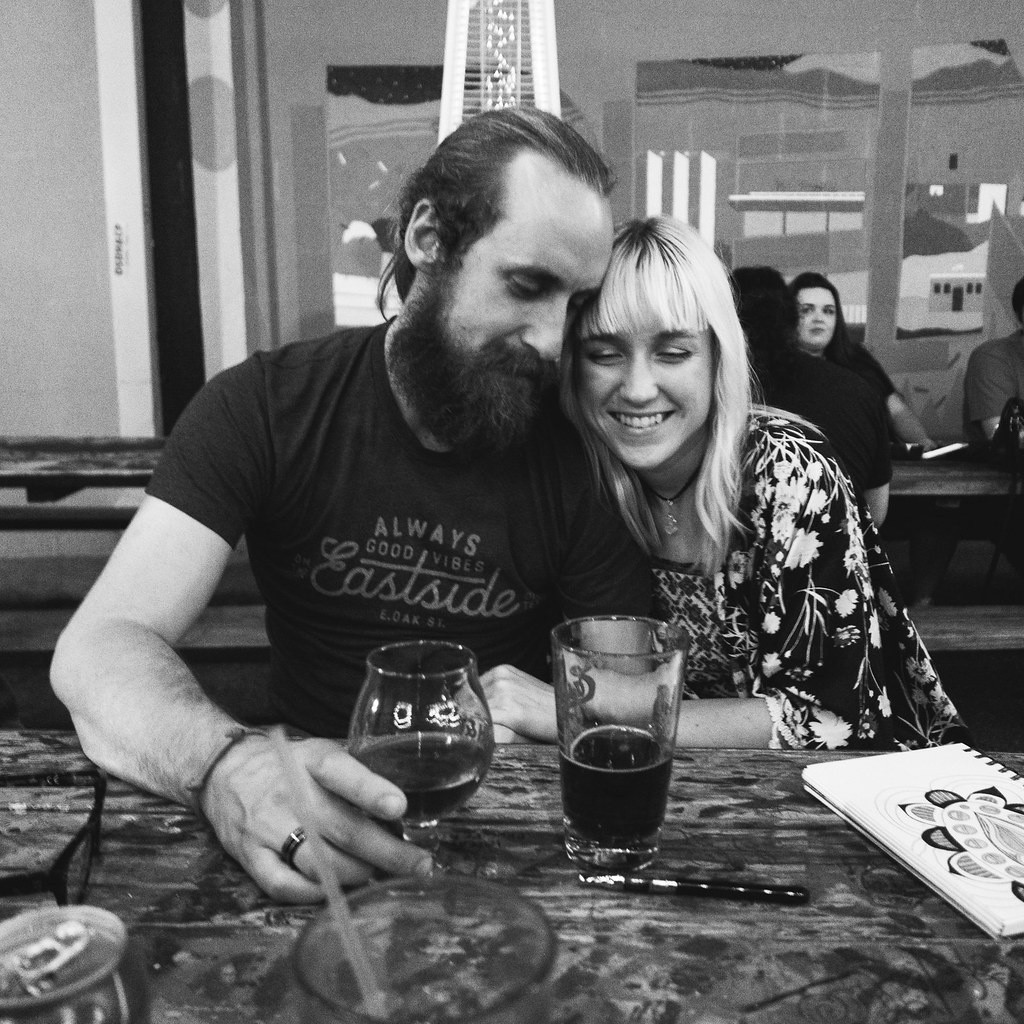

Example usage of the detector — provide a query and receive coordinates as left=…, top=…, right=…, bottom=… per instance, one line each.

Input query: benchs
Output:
left=0, top=503, right=1024, bottom=711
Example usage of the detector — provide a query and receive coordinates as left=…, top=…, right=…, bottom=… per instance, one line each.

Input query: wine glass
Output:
left=350, top=644, right=496, bottom=902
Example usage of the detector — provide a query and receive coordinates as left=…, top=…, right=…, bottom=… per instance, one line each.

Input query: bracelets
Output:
left=186, top=724, right=269, bottom=825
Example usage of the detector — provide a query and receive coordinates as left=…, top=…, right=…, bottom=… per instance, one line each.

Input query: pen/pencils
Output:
left=578, top=872, right=810, bottom=905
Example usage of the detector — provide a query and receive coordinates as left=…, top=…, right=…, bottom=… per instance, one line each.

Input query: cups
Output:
left=551, top=615, right=691, bottom=873
left=293, top=873, right=561, bottom=1023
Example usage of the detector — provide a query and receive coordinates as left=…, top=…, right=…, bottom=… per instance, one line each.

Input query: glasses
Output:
left=0, top=768, right=107, bottom=904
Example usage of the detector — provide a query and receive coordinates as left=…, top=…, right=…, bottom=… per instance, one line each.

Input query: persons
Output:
left=45, top=106, right=675, bottom=907
left=964, top=277, right=1024, bottom=443
left=446, top=214, right=971, bottom=754
left=728, top=265, right=959, bottom=606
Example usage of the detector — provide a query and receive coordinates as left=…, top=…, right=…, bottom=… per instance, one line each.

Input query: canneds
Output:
left=0, top=903, right=152, bottom=1024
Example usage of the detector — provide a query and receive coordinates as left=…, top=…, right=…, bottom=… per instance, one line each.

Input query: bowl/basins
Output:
left=895, top=442, right=923, bottom=457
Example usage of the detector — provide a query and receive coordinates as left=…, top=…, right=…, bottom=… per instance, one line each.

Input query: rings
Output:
left=279, top=826, right=308, bottom=872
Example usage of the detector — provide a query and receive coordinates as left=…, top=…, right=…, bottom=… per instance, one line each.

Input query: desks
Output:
left=0, top=725, right=1024, bottom=1024
left=0, top=428, right=221, bottom=508
left=844, top=432, right=1024, bottom=606
